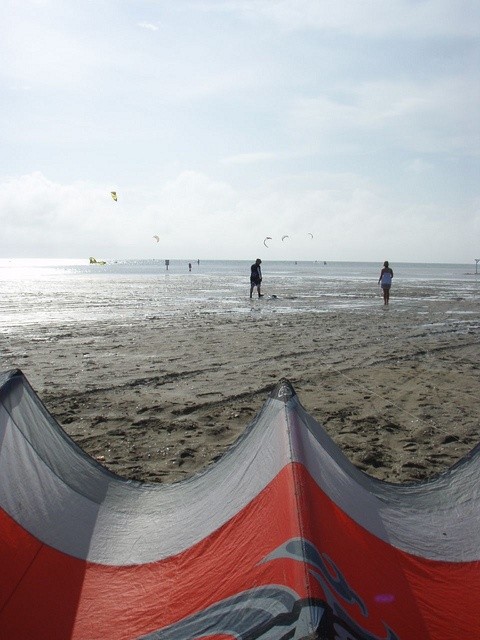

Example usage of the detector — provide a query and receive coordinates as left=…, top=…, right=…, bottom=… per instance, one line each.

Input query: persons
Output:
left=378, top=260, right=393, bottom=305
left=249, top=259, right=264, bottom=298
